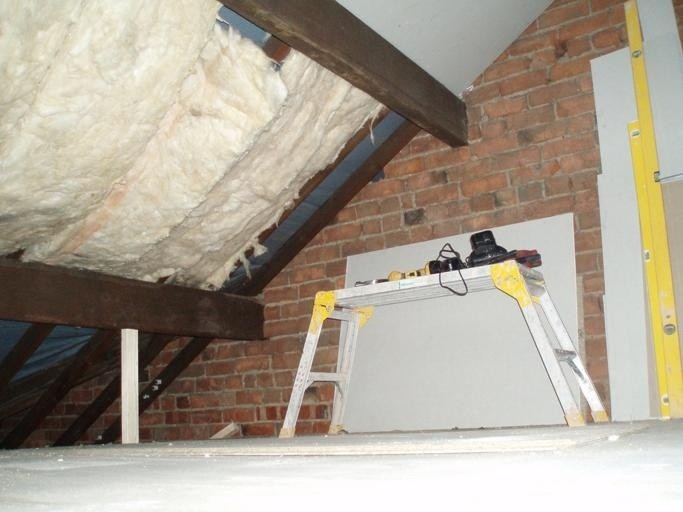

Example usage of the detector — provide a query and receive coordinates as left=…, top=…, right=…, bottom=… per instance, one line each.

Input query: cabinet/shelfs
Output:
left=278, top=258, right=609, bottom=439
